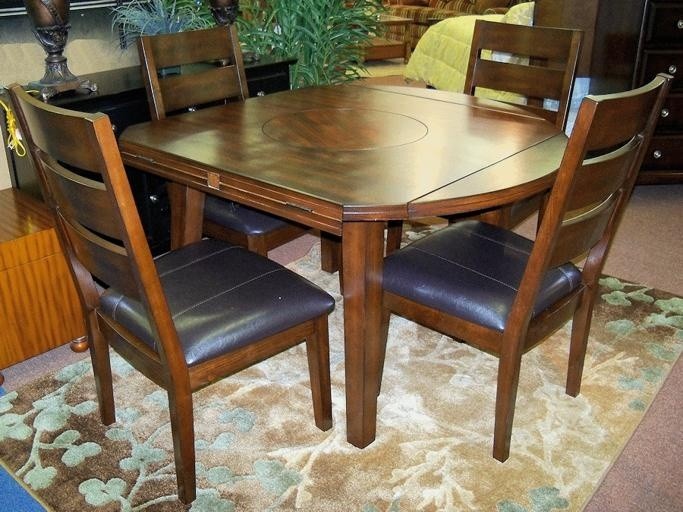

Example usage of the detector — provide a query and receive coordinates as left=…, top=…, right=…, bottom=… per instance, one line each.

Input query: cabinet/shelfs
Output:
left=0, top=52, right=300, bottom=291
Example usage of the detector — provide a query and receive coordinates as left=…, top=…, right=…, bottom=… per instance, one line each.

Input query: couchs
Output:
left=344, top=0, right=519, bottom=50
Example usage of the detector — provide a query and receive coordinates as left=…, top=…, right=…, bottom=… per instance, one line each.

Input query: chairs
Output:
left=377, top=65, right=674, bottom=461
left=386, top=15, right=586, bottom=256
left=132, top=24, right=336, bottom=276
left=6, top=81, right=337, bottom=503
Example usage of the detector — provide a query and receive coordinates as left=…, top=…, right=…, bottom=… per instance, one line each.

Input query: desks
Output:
left=109, top=76, right=565, bottom=451
left=348, top=10, right=414, bottom=64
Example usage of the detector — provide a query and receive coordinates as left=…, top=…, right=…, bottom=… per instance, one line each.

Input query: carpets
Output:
left=0, top=215, right=682, bottom=512
